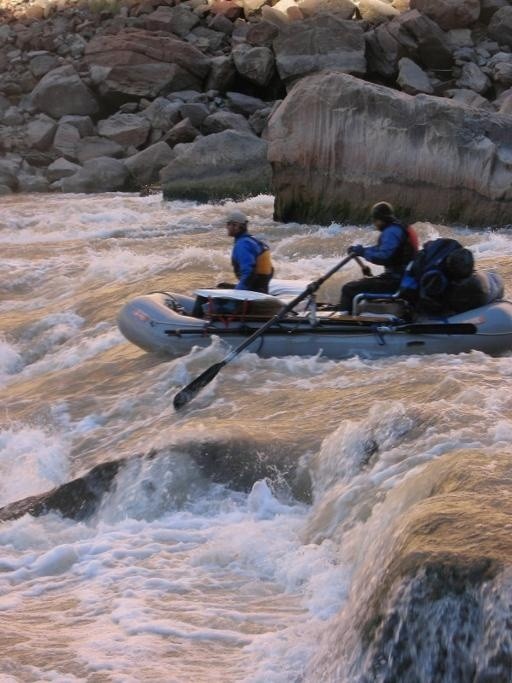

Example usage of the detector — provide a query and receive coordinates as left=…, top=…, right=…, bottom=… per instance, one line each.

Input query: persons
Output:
left=338, top=201, right=418, bottom=311
left=192, top=212, right=274, bottom=319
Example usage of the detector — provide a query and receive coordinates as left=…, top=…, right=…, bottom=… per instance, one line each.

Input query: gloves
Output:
left=352, top=245, right=363, bottom=255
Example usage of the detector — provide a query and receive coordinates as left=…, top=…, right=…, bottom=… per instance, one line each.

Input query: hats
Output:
left=371, top=201, right=395, bottom=221
left=223, top=211, right=248, bottom=224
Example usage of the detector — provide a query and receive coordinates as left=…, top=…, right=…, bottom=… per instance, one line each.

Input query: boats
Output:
left=118, top=279, right=512, bottom=359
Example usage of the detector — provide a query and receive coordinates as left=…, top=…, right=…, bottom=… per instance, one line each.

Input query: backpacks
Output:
left=399, top=238, right=473, bottom=315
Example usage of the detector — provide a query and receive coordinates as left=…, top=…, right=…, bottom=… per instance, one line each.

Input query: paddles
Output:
left=174, top=250, right=357, bottom=409
left=164, top=323, right=476, bottom=335
